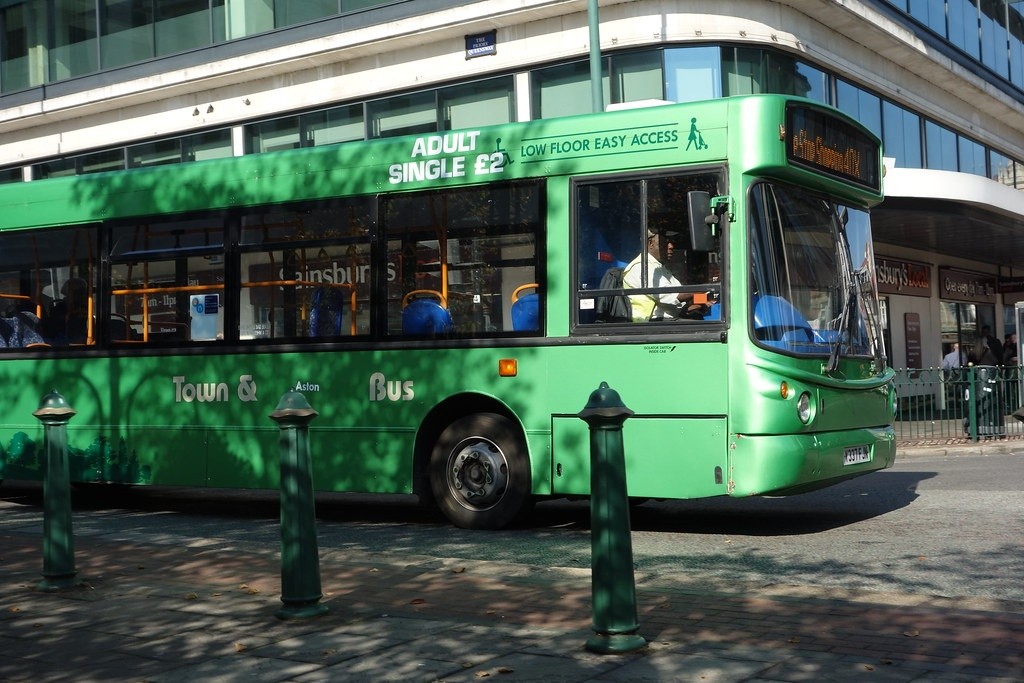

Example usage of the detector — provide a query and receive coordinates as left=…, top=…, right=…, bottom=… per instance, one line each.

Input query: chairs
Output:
left=0, top=268, right=629, bottom=352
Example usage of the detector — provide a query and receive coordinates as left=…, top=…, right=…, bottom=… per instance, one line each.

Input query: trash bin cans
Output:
left=963, top=366, right=1005, bottom=434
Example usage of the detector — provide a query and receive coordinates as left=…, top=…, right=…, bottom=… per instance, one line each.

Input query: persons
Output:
left=53, top=278, right=88, bottom=338
left=622, top=225, right=717, bottom=322
left=944, top=343, right=968, bottom=381
left=981, top=325, right=1017, bottom=366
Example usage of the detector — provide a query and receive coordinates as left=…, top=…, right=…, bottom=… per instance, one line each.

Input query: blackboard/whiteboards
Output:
left=905, top=313, right=921, bottom=379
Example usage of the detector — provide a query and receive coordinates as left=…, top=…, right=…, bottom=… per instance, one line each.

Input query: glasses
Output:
left=660, top=236, right=679, bottom=244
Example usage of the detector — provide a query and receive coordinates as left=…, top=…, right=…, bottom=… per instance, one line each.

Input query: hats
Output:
left=645, top=221, right=680, bottom=239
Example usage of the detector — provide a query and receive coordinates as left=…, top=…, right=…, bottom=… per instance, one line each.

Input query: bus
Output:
left=0, top=92, right=902, bottom=535
